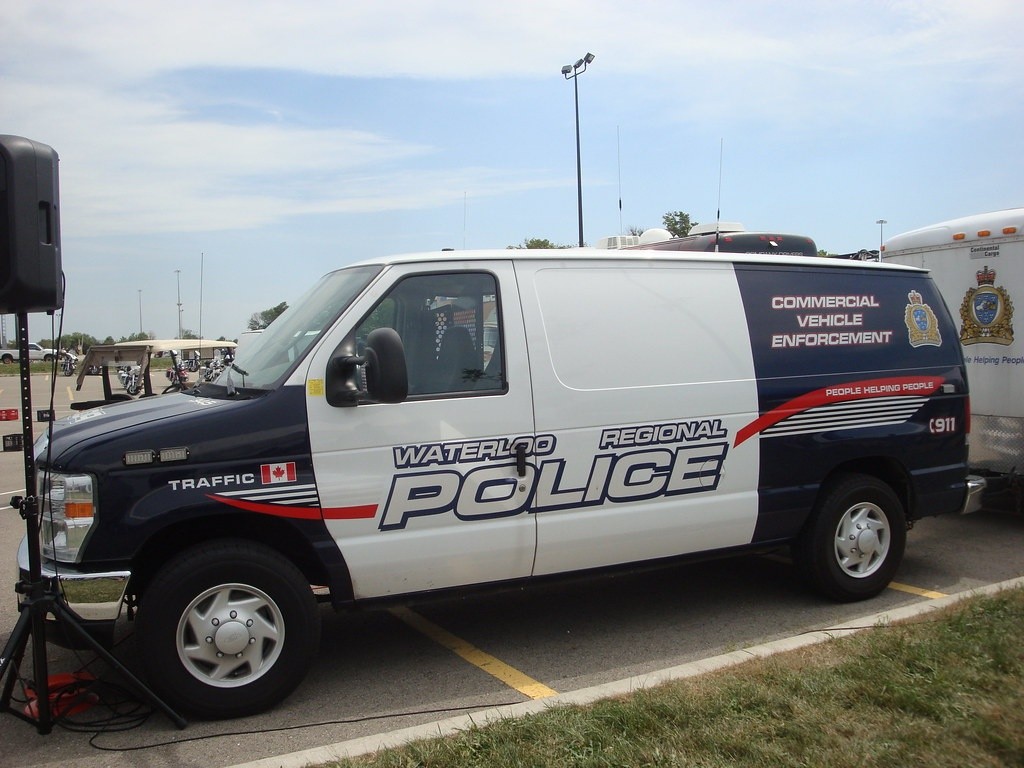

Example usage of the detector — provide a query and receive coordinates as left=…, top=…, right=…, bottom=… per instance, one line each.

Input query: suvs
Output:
left=20, top=244, right=974, bottom=724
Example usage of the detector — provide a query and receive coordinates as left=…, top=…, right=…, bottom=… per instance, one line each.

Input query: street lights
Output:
left=174, top=268, right=184, bottom=339
left=561, top=51, right=597, bottom=247
left=876, top=219, right=888, bottom=264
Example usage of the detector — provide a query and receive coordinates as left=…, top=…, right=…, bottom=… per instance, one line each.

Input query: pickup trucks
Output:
left=0, top=341, right=60, bottom=365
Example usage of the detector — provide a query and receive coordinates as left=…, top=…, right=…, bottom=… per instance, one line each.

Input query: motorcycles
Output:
left=58, top=349, right=235, bottom=397
